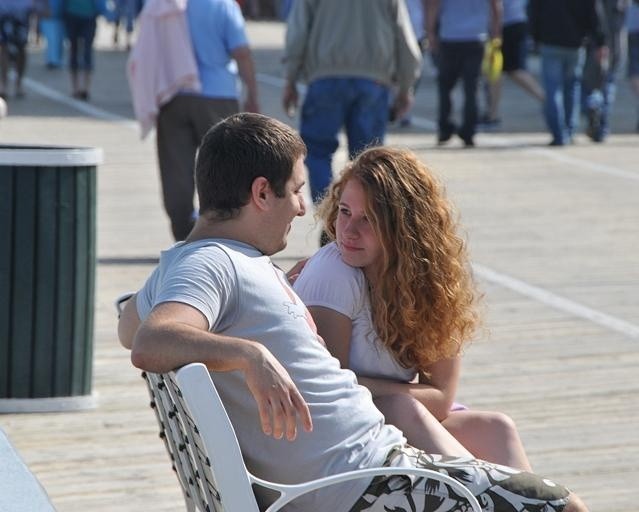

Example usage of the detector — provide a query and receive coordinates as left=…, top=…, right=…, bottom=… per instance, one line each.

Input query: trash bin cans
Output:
left=0, top=144, right=101, bottom=414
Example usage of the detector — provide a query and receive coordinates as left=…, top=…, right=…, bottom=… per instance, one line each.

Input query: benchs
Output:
left=114, top=293, right=485, bottom=511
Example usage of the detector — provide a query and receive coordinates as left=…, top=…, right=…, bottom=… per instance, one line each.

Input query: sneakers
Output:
left=474, top=113, right=504, bottom=133
left=586, top=107, right=601, bottom=137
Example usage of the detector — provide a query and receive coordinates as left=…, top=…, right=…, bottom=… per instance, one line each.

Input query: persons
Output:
left=290, top=140, right=533, bottom=476
left=0, top=0, right=146, bottom=116
left=275, top=1, right=639, bottom=147
left=280, top=1, right=426, bottom=202
left=119, top=110, right=587, bottom=511
left=141, top=0, right=259, bottom=246
